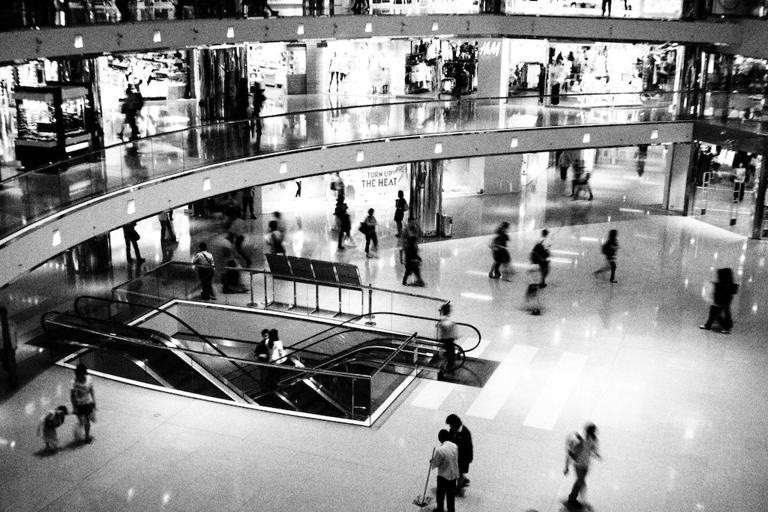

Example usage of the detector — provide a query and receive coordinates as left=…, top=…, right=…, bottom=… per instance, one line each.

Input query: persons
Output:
left=254, top=328, right=289, bottom=391
left=435, top=304, right=458, bottom=371
left=550, top=61, right=561, bottom=105
left=731, top=162, right=747, bottom=203
left=557, top=150, right=593, bottom=201
left=699, top=267, right=737, bottom=335
left=429, top=429, right=459, bottom=512
left=331, top=171, right=426, bottom=287
left=562, top=423, right=602, bottom=512
left=251, top=81, right=262, bottom=118
left=75, top=186, right=290, bottom=300
left=36, top=363, right=96, bottom=451
left=593, top=229, right=619, bottom=283
left=445, top=414, right=473, bottom=489
left=487, top=220, right=552, bottom=315
left=115, top=89, right=139, bottom=141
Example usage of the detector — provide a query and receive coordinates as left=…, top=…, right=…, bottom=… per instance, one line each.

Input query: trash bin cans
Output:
left=440, top=214, right=453, bottom=238
